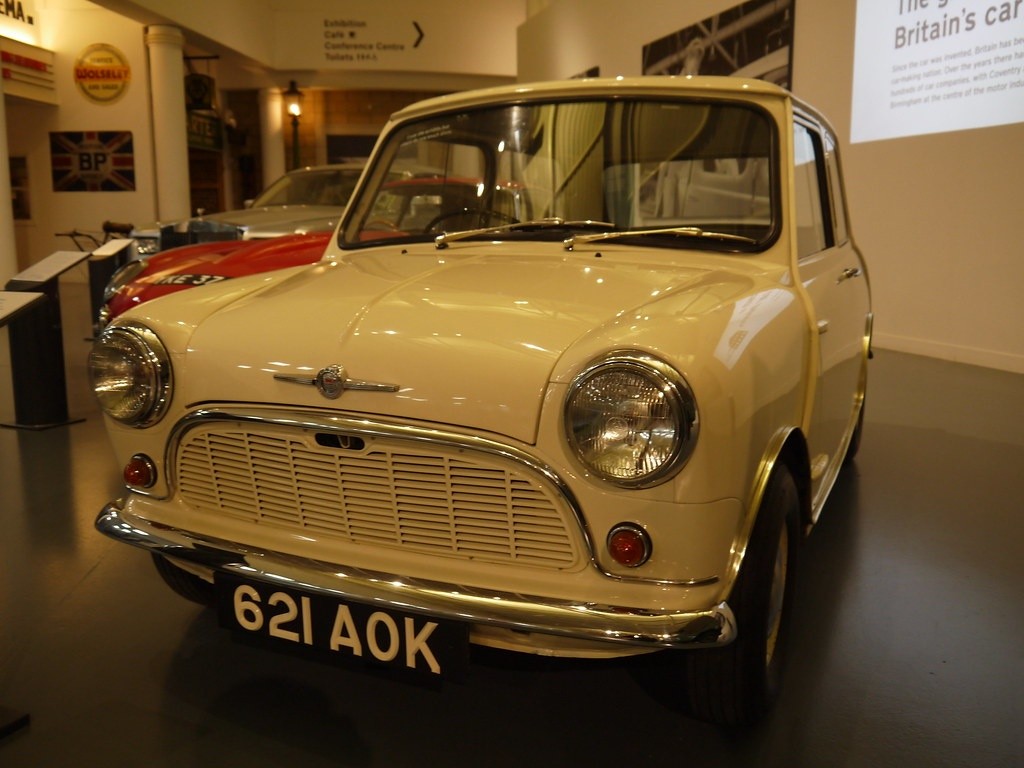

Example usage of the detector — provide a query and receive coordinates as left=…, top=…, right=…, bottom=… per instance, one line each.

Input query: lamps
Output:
left=283, top=80, right=304, bottom=117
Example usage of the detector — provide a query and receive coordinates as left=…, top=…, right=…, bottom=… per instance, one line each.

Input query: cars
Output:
left=85, top=72, right=875, bottom=731
left=93, top=176, right=537, bottom=352
left=128, top=161, right=454, bottom=267
